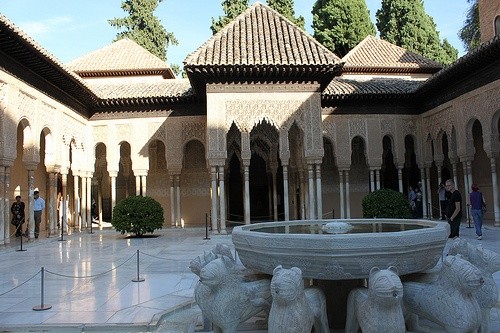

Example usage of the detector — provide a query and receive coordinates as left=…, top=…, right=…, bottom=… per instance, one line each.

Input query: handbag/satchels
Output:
left=482, top=203, right=488, bottom=213
left=11, top=217, right=16, bottom=225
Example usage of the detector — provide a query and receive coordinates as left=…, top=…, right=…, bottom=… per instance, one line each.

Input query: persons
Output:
left=437, top=179, right=463, bottom=238
left=91, top=195, right=99, bottom=220
left=11, top=196, right=25, bottom=237
left=22, top=191, right=45, bottom=238
left=467, top=182, right=486, bottom=240
left=409, top=184, right=423, bottom=218
left=59, top=196, right=71, bottom=229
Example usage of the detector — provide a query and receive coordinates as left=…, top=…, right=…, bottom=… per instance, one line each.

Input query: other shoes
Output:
left=476, top=234, right=482, bottom=240
